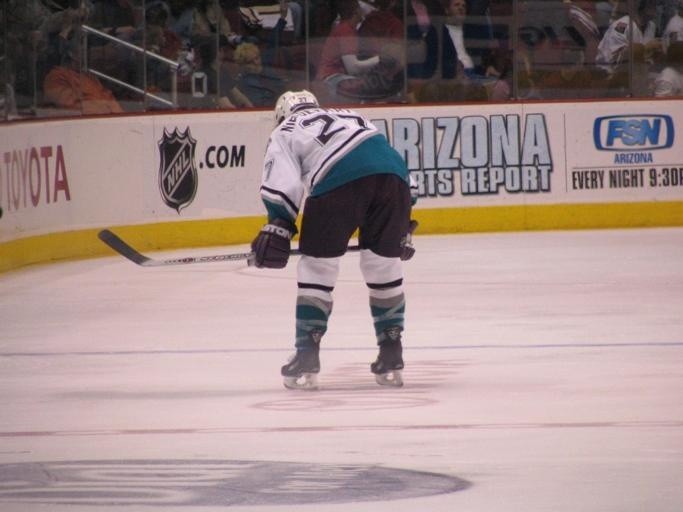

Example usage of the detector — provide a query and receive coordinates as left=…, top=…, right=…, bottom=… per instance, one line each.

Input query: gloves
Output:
left=399, top=219, right=418, bottom=262
left=249, top=216, right=298, bottom=270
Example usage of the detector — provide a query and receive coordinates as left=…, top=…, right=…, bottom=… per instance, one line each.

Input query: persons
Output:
left=2, top=0, right=683, bottom=118
left=246, top=87, right=418, bottom=387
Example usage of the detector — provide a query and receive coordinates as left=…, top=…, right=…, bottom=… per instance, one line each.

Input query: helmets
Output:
left=274, top=89, right=320, bottom=124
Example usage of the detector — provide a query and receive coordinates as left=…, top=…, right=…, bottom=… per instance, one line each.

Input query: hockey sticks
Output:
left=97, top=228, right=361, bottom=266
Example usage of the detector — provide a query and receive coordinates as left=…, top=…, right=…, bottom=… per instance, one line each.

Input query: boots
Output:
left=370, top=327, right=404, bottom=373
left=280, top=331, right=322, bottom=375
